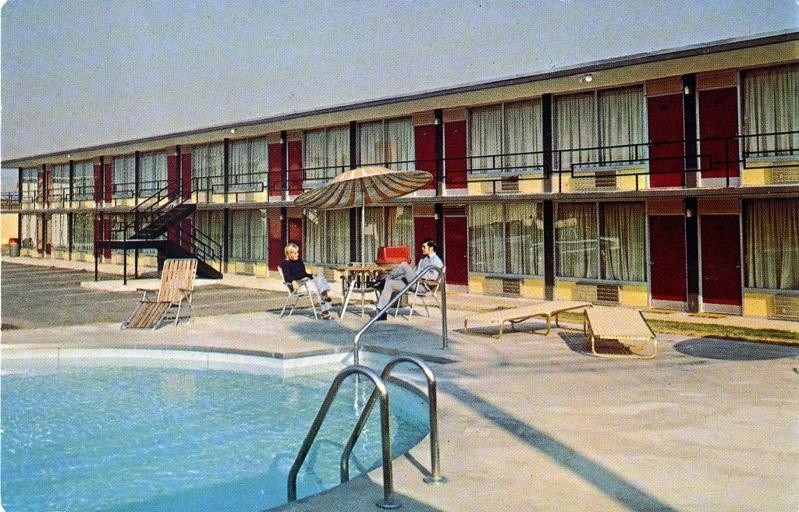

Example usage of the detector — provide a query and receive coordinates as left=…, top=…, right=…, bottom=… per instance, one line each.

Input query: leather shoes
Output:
left=374, top=311, right=387, bottom=321
left=368, top=279, right=385, bottom=291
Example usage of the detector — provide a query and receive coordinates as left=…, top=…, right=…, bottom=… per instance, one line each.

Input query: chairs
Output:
left=119, top=256, right=197, bottom=333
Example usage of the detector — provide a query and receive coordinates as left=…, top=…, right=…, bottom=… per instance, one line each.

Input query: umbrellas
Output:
left=293, top=166, right=433, bottom=319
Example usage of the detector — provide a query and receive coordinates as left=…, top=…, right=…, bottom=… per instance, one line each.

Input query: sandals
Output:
left=321, top=311, right=337, bottom=321
left=320, top=295, right=332, bottom=305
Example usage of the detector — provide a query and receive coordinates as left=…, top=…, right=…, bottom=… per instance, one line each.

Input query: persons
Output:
left=368, top=240, right=445, bottom=320
left=280, top=243, right=335, bottom=320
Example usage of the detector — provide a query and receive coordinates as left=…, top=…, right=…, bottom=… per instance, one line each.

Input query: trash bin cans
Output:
left=374, top=245, right=413, bottom=308
left=9, top=238, right=20, bottom=257
